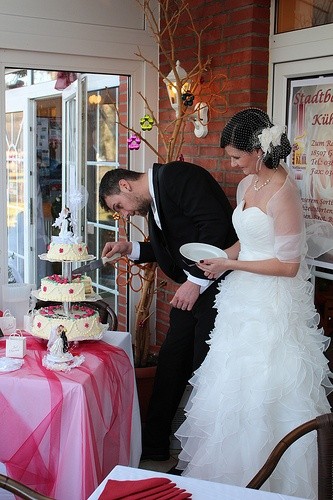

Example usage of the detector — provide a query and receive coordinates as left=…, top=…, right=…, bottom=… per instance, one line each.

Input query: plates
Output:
left=179, top=243, right=228, bottom=263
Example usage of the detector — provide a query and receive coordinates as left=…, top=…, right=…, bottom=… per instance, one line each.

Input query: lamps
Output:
left=161, top=60, right=207, bottom=139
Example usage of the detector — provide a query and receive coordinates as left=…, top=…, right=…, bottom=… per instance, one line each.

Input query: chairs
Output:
left=245, top=412, right=333, bottom=500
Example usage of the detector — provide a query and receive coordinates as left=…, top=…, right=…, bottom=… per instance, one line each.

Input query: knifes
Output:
left=72, top=253, right=121, bottom=274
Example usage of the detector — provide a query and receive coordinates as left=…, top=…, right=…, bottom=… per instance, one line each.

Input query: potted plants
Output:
left=98, top=0, right=224, bottom=421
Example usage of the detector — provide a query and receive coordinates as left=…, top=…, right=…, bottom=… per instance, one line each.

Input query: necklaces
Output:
left=254, top=167, right=278, bottom=192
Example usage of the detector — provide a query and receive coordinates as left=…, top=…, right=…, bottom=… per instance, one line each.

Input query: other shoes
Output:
left=166, top=464, right=184, bottom=476
left=142, top=448, right=171, bottom=461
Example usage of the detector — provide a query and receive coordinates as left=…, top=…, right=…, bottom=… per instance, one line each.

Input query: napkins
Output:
left=95, top=477, right=192, bottom=500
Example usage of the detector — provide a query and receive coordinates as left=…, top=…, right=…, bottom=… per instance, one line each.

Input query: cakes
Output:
left=30, top=305, right=103, bottom=341
left=45, top=208, right=90, bottom=261
left=35, top=274, right=92, bottom=301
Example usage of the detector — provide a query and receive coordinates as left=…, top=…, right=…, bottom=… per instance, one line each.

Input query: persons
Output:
left=174, top=108, right=333, bottom=500
left=99, top=161, right=239, bottom=461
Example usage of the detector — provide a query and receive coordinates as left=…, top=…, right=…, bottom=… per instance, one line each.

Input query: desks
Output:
left=0, top=332, right=142, bottom=500
left=87, top=466, right=309, bottom=500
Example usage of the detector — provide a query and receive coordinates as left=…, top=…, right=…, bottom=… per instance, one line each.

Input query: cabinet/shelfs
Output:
left=29, top=211, right=107, bottom=342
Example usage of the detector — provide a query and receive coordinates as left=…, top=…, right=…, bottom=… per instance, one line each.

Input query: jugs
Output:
left=1, top=283, right=37, bottom=331
